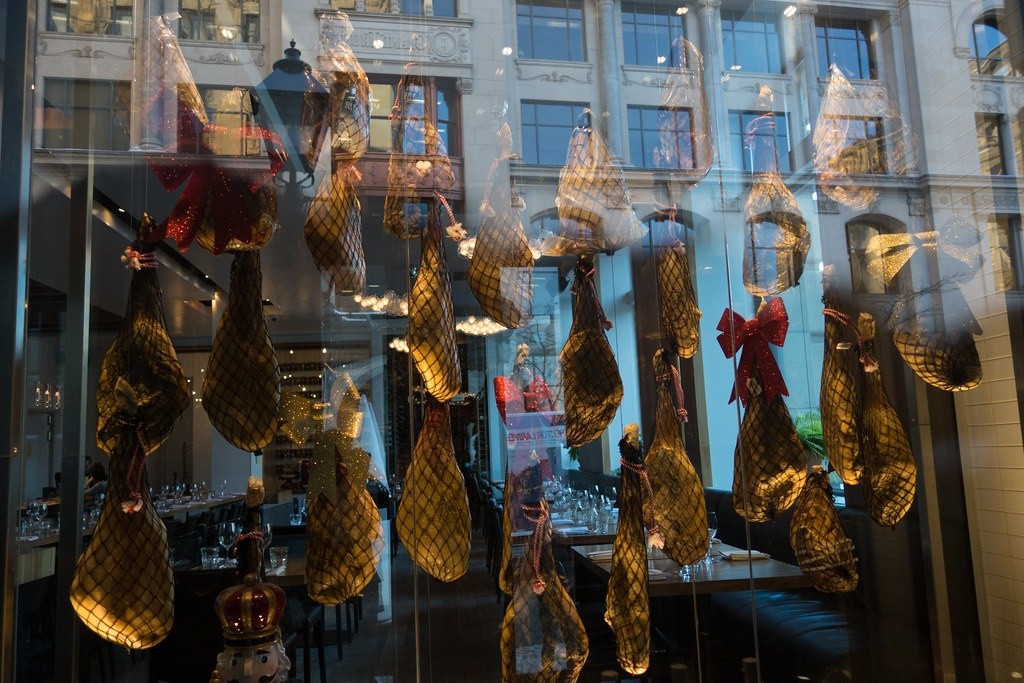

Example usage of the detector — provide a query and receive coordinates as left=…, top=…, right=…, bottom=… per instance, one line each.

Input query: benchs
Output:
left=565, top=468, right=870, bottom=683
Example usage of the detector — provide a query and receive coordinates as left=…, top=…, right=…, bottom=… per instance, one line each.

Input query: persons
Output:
left=801, top=439, right=833, bottom=503
left=83, top=462, right=108, bottom=515
left=85, top=455, right=96, bottom=488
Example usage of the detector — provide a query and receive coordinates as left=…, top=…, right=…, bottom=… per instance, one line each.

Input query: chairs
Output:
left=158, top=501, right=246, bottom=551
left=474, top=480, right=676, bottom=683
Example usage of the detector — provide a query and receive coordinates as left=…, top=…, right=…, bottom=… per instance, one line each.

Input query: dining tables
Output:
left=570, top=539, right=811, bottom=683
left=153, top=529, right=324, bottom=683
left=18, top=477, right=248, bottom=546
left=549, top=509, right=619, bottom=561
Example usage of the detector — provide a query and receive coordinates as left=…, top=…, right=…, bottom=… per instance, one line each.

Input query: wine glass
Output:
left=262, top=523, right=272, bottom=560
left=25, top=497, right=48, bottom=543
left=701, top=512, right=720, bottom=564
left=551, top=479, right=617, bottom=524
left=218, top=522, right=236, bottom=568
left=94, top=480, right=227, bottom=512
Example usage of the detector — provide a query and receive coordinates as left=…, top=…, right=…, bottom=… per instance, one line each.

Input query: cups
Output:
left=269, top=547, right=289, bottom=571
left=22, top=509, right=100, bottom=536
left=200, top=547, right=220, bottom=568
left=552, top=495, right=608, bottom=534
left=290, top=514, right=302, bottom=526
left=682, top=565, right=700, bottom=578
